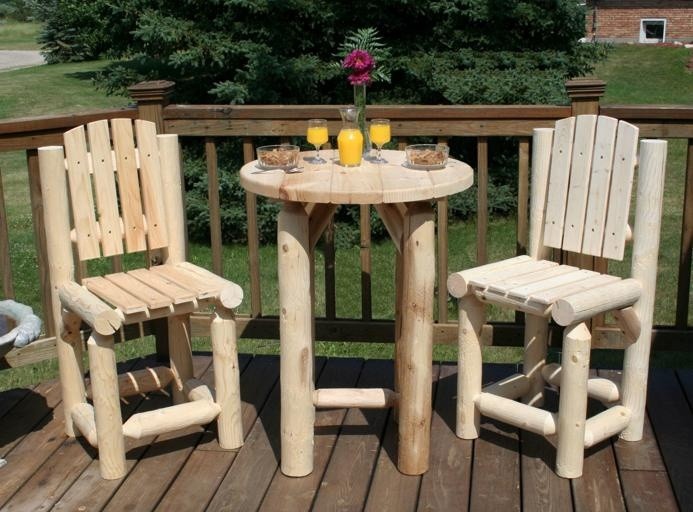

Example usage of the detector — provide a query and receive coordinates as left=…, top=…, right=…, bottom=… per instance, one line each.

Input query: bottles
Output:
left=337, top=107, right=364, bottom=167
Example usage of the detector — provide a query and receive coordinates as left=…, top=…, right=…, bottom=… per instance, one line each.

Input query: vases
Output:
left=352, top=84, right=371, bottom=154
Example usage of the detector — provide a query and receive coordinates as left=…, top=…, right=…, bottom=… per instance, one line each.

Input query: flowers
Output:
left=322, top=27, right=392, bottom=147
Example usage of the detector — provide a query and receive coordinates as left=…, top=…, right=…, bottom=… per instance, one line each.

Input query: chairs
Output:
left=446, top=113, right=669, bottom=483
left=35, top=117, right=247, bottom=481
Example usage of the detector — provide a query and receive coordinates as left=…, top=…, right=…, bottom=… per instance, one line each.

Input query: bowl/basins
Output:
left=405, top=144, right=451, bottom=170
left=255, top=144, right=300, bottom=170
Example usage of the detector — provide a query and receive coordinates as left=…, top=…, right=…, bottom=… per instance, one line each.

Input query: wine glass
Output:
left=306, top=119, right=329, bottom=165
left=370, top=119, right=392, bottom=164
